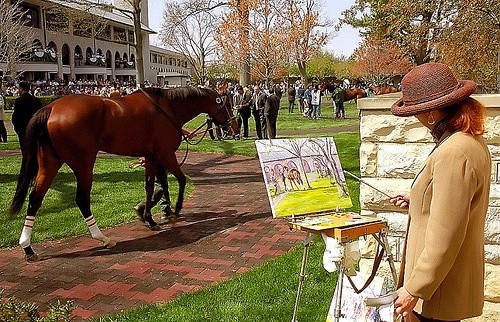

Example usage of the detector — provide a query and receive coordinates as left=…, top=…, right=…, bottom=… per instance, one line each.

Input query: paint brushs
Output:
left=344, top=170, right=393, bottom=199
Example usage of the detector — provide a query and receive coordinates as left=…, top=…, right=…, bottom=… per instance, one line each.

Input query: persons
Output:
left=310, top=84, right=325, bottom=119
left=389, top=61, right=492, bottom=322
left=197, top=81, right=306, bottom=140
left=1, top=79, right=168, bottom=96
left=11, top=81, right=43, bottom=155
left=0, top=91, right=7, bottom=142
left=331, top=80, right=402, bottom=119
left=132, top=156, right=179, bottom=226
left=303, top=83, right=313, bottom=120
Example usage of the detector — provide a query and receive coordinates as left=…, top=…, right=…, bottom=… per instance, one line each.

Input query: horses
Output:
left=321, top=79, right=366, bottom=120
left=9, top=85, right=239, bottom=263
left=369, top=85, right=399, bottom=96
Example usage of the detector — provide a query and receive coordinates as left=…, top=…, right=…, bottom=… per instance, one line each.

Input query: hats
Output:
left=391, top=63, right=476, bottom=117
